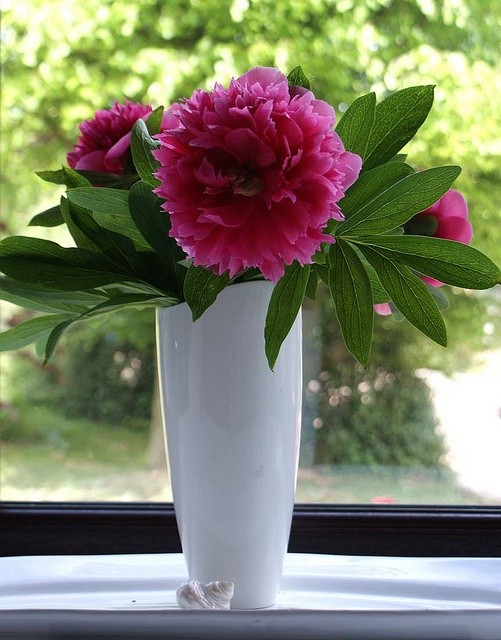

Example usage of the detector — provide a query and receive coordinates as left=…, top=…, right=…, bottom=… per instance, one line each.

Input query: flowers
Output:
left=0, top=65, right=501, bottom=371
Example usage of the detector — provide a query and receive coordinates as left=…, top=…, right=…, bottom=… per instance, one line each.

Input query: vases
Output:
left=154, top=278, right=304, bottom=610
